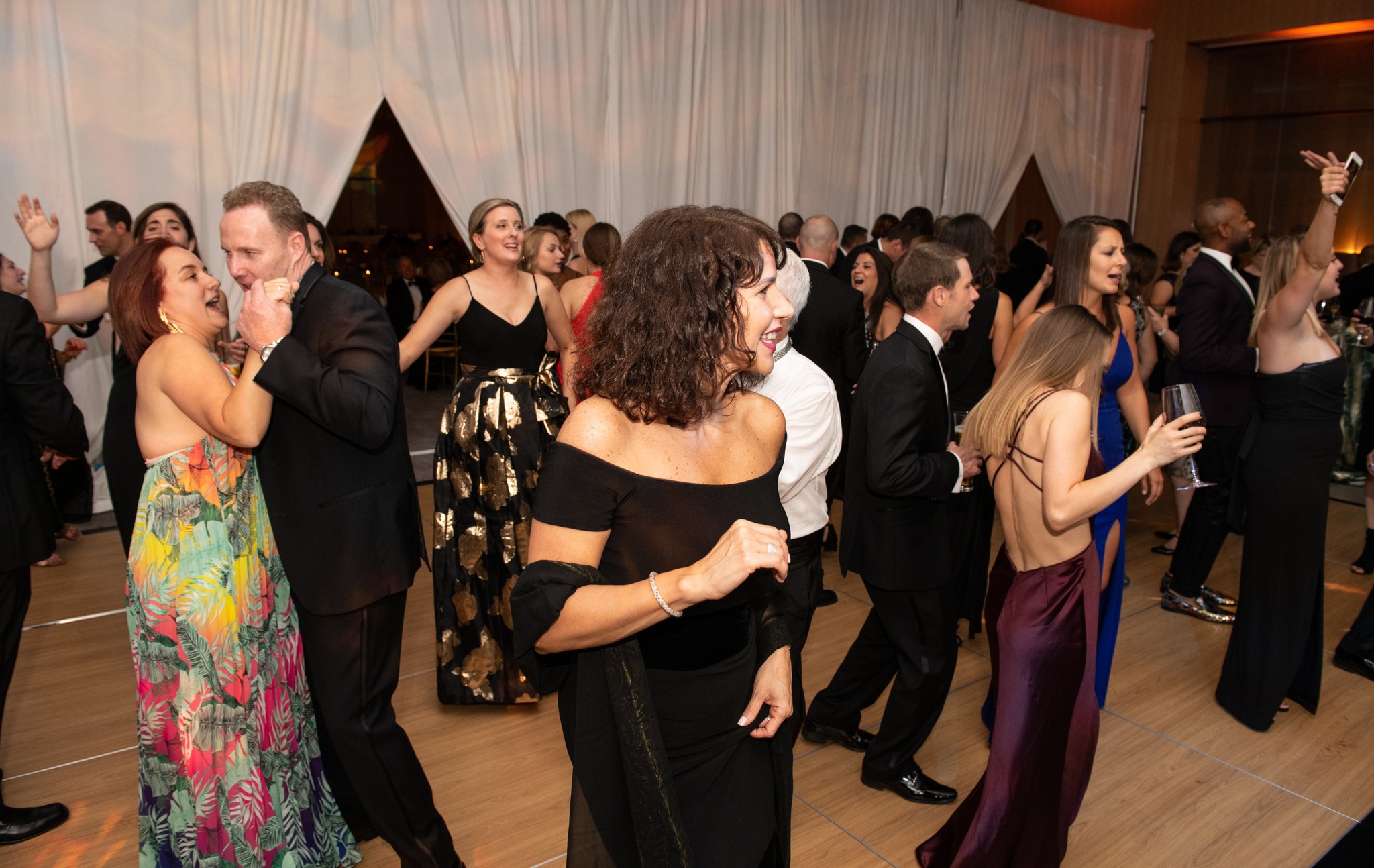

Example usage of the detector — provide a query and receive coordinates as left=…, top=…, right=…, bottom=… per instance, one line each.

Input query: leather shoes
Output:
left=801, top=717, right=875, bottom=751
left=861, top=753, right=958, bottom=804
left=1161, top=573, right=1238, bottom=606
left=0, top=802, right=69, bottom=846
left=816, top=589, right=837, bottom=607
left=1161, top=590, right=1236, bottom=624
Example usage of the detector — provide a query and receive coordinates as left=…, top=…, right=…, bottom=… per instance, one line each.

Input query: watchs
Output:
left=259, top=335, right=286, bottom=364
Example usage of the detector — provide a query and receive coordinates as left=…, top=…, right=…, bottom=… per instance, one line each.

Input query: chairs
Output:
left=422, top=322, right=463, bottom=396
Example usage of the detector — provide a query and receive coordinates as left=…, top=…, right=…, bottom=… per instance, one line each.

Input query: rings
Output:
left=244, top=343, right=248, bottom=351
left=768, top=714, right=771, bottom=721
left=278, top=285, right=283, bottom=291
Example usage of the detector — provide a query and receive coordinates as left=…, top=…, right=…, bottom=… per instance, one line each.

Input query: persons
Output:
left=511, top=204, right=795, bottom=866
left=109, top=235, right=362, bottom=868
left=914, top=304, right=1208, bottom=866
left=778, top=142, right=1371, bottom=734
left=0, top=191, right=626, bottom=568
left=391, top=197, right=582, bottom=709
left=220, top=179, right=473, bottom=867
left=980, top=215, right=1163, bottom=748
left=1, top=287, right=94, bottom=848
left=802, top=244, right=982, bottom=804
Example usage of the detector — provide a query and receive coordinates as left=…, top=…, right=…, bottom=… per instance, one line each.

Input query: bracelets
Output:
left=649, top=571, right=683, bottom=618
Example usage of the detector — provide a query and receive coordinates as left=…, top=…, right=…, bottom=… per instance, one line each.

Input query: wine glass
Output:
left=1346, top=298, right=1374, bottom=348
left=1161, top=382, right=1217, bottom=490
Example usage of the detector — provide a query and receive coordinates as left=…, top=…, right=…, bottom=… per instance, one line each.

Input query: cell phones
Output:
left=1330, top=151, right=1363, bottom=206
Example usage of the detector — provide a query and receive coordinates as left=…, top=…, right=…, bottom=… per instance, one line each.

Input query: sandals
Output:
left=1350, top=528, right=1374, bottom=575
left=34, top=552, right=67, bottom=567
left=64, top=524, right=81, bottom=540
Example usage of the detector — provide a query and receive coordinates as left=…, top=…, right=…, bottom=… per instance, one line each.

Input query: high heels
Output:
left=1155, top=531, right=1176, bottom=539
left=1151, top=536, right=1178, bottom=555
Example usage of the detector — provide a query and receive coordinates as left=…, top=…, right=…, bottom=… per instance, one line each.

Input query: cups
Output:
left=1149, top=294, right=1165, bottom=319
left=952, top=409, right=975, bottom=492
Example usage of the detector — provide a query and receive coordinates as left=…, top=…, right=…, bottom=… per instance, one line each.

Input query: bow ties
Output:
left=1231, top=255, right=1243, bottom=269
left=408, top=282, right=418, bottom=287
